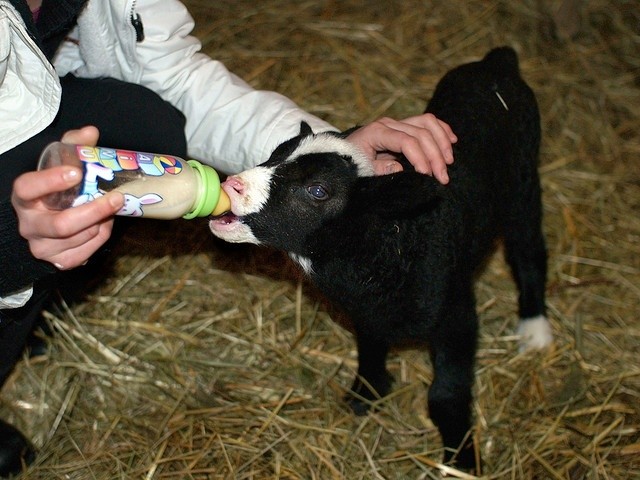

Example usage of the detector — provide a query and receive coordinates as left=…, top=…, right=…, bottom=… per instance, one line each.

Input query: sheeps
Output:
left=209, top=45, right=549, bottom=480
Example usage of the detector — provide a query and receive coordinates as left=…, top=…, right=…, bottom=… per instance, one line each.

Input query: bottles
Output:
left=38, top=142, right=231, bottom=221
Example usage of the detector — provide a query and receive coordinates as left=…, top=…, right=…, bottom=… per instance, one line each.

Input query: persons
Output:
left=1, top=1, right=458, bottom=480
